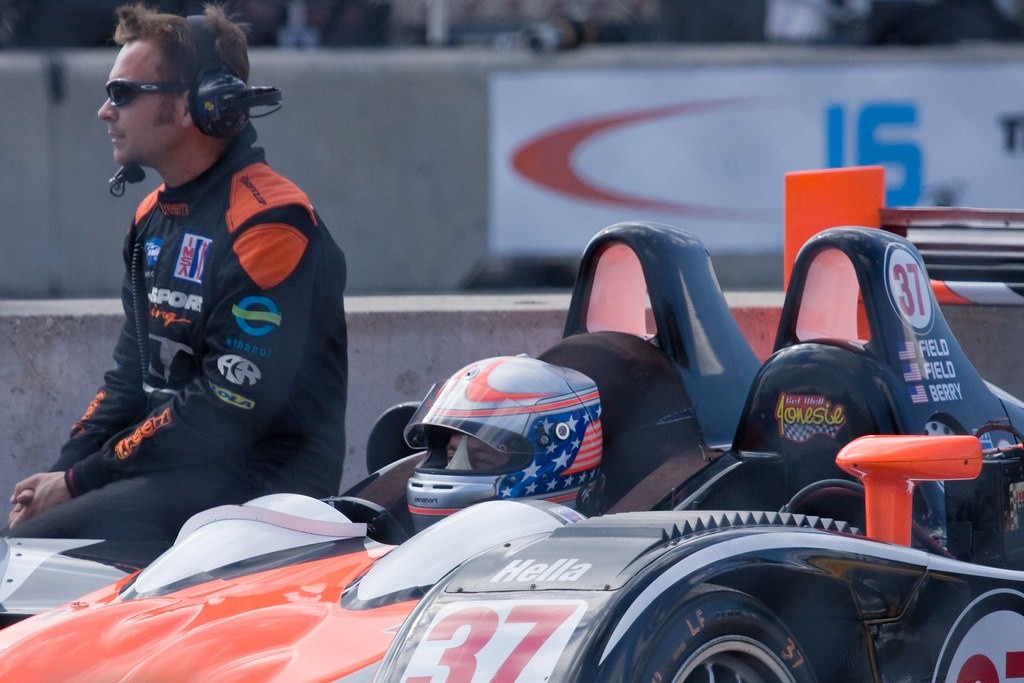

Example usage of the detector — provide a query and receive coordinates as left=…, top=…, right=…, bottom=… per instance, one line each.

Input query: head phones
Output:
left=187, top=74, right=280, bottom=137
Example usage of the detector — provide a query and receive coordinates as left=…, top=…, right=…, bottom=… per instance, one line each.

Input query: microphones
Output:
left=109, top=165, right=145, bottom=187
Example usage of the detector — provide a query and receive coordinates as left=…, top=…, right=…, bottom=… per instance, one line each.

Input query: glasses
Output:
left=105, top=78, right=188, bottom=106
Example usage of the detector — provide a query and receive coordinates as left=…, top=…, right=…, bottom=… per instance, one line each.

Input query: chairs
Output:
left=343, top=331, right=726, bottom=545
left=670, top=337, right=947, bottom=556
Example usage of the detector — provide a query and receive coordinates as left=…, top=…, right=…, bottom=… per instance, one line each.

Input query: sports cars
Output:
left=0, top=221, right=1024, bottom=683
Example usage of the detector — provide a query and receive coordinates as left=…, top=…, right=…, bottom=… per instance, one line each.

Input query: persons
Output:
left=0, top=5, right=349, bottom=539
left=404, top=350, right=606, bottom=533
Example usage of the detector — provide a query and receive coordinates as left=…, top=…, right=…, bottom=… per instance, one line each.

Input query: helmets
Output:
left=402, top=354, right=602, bottom=536
